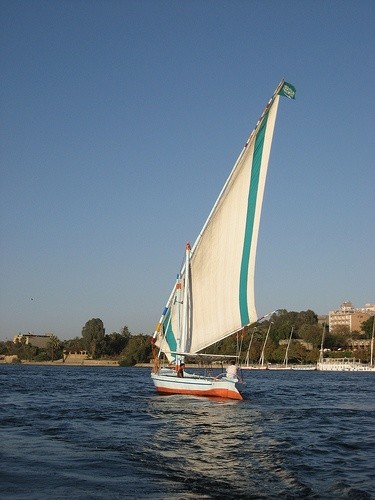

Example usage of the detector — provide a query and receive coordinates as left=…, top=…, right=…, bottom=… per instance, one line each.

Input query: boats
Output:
left=317, top=358, right=375, bottom=372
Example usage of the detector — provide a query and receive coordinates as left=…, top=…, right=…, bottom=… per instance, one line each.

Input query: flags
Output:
left=278, top=81, right=298, bottom=100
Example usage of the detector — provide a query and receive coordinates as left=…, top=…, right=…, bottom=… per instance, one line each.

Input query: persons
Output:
left=177, top=363, right=185, bottom=378
left=226, top=361, right=239, bottom=380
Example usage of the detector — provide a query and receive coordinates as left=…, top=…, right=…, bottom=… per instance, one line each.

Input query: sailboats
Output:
left=151, top=78, right=299, bottom=400
